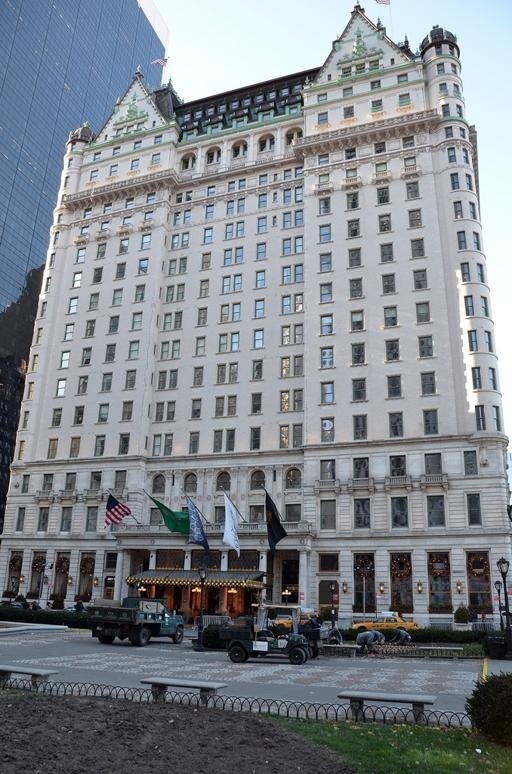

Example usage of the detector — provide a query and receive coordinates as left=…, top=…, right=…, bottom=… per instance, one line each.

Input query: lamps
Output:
left=341, top=580, right=463, bottom=595
left=16, top=573, right=101, bottom=587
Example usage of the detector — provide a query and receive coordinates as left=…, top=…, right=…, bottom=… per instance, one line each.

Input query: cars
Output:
left=350, top=614, right=418, bottom=632
left=1, top=600, right=36, bottom=609
left=275, top=611, right=320, bottom=630
left=47, top=605, right=87, bottom=613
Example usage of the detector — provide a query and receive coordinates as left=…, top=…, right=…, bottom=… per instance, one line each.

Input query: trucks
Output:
left=216, top=604, right=313, bottom=665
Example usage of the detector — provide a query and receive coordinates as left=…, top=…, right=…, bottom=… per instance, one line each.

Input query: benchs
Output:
left=339, top=691, right=438, bottom=723
left=419, top=646, right=463, bottom=659
left=144, top=677, right=226, bottom=706
left=1, top=662, right=57, bottom=691
left=316, top=643, right=363, bottom=657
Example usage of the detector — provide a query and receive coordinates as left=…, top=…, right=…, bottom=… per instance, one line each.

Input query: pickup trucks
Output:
left=87, top=596, right=185, bottom=644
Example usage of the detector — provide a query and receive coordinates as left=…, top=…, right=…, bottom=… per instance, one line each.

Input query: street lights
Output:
left=329, top=580, right=338, bottom=628
left=226, top=587, right=238, bottom=617
left=193, top=564, right=206, bottom=651
left=36, top=563, right=53, bottom=604
left=138, top=586, right=147, bottom=599
left=494, top=580, right=504, bottom=632
left=9, top=574, right=17, bottom=602
left=192, top=586, right=201, bottom=615
left=496, top=556, right=512, bottom=660
left=281, top=589, right=292, bottom=606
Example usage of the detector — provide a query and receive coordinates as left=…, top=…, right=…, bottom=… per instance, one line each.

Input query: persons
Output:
left=356, top=632, right=382, bottom=658
left=326, top=628, right=344, bottom=646
left=391, top=629, right=412, bottom=646
left=303, top=617, right=323, bottom=629
left=75, top=596, right=80, bottom=601
left=372, top=630, right=385, bottom=643
left=74, top=601, right=84, bottom=613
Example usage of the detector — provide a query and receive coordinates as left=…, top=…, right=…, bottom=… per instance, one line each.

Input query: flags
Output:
left=150, top=58, right=168, bottom=67
left=222, top=492, right=241, bottom=560
left=102, top=494, right=131, bottom=529
left=144, top=490, right=190, bottom=534
left=375, top=1, right=391, bottom=6
left=184, top=495, right=210, bottom=557
left=263, top=491, right=289, bottom=552
